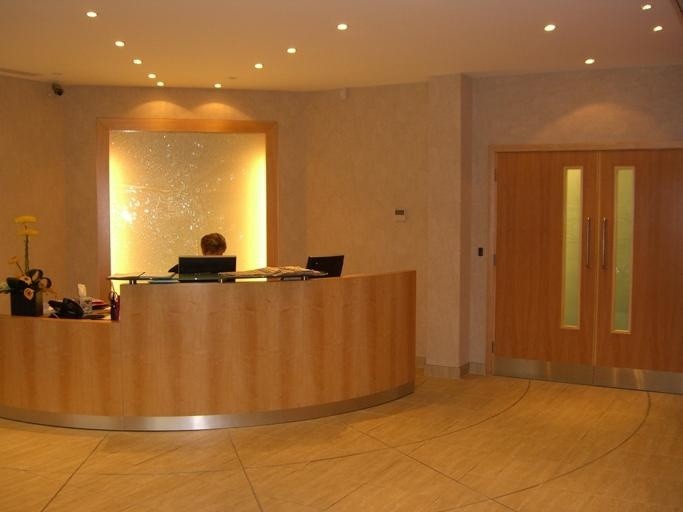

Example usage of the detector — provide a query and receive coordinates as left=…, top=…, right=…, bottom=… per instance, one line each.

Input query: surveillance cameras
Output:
left=52, top=84, right=64, bottom=95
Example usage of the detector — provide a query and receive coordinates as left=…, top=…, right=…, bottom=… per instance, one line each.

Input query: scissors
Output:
left=109, top=291, right=119, bottom=306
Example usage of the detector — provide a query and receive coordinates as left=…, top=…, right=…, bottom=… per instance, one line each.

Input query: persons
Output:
left=201, top=233, right=228, bottom=256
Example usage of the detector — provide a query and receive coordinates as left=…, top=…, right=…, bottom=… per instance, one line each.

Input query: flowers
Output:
left=0, top=215, right=52, bottom=300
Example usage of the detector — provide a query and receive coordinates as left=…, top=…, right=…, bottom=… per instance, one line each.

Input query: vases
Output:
left=10, top=286, right=43, bottom=316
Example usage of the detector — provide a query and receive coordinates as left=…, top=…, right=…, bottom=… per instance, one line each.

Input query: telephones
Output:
left=48, top=298, right=83, bottom=317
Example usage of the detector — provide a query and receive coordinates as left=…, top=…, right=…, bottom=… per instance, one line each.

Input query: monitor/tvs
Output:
left=179, top=256, right=236, bottom=282
left=306, top=255, right=344, bottom=278
